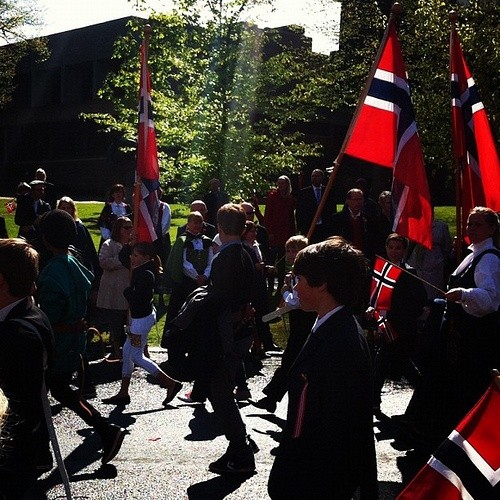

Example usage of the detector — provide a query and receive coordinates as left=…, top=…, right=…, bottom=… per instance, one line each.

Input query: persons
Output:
left=1, top=168, right=500, bottom=500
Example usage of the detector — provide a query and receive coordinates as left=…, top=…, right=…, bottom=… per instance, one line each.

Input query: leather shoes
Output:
left=247, top=397, right=277, bottom=413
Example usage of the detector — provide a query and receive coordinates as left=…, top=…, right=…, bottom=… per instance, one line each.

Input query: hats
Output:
left=29, top=180, right=45, bottom=184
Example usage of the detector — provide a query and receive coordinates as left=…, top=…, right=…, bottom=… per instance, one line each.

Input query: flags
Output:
left=135, top=33, right=162, bottom=244
left=371, top=255, right=401, bottom=340
left=450, top=26, right=500, bottom=235
left=397, top=380, right=500, bottom=500
left=343, top=28, right=433, bottom=248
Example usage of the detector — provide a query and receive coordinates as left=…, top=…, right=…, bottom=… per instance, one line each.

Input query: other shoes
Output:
left=162, top=381, right=183, bottom=405
left=101, top=395, right=130, bottom=405
left=208, top=445, right=255, bottom=477
left=101, top=425, right=125, bottom=465
